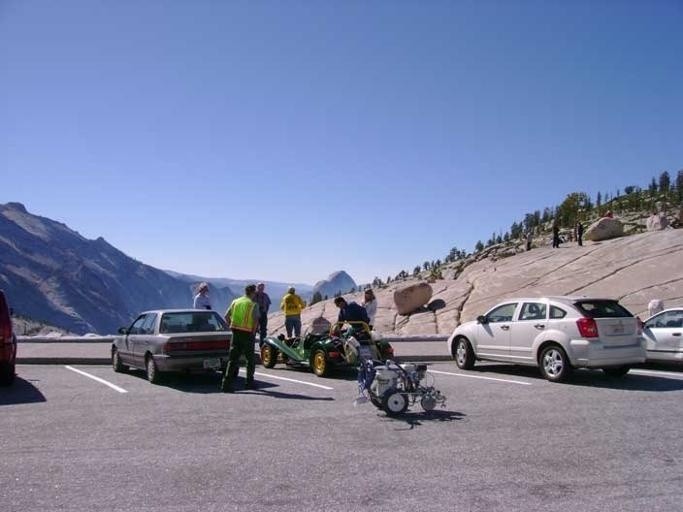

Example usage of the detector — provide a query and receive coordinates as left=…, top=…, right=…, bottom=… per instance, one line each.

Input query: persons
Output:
left=280, top=287, right=305, bottom=337
left=252, top=283, right=271, bottom=348
left=604, top=209, right=613, bottom=218
left=334, top=297, right=371, bottom=331
left=220, top=284, right=261, bottom=393
left=577, top=222, right=584, bottom=246
left=192, top=281, right=212, bottom=324
left=552, top=223, right=560, bottom=248
left=526, top=231, right=533, bottom=250
left=361, top=289, right=378, bottom=331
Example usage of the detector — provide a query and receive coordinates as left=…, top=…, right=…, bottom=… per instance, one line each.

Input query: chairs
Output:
left=522, top=303, right=542, bottom=320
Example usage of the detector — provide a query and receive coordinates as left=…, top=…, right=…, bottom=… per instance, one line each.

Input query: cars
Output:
left=110, top=308, right=239, bottom=384
left=446, top=295, right=646, bottom=382
left=260, top=320, right=394, bottom=376
left=642, top=307, right=683, bottom=365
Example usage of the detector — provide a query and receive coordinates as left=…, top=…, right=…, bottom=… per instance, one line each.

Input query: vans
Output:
left=0, top=289, right=17, bottom=383
